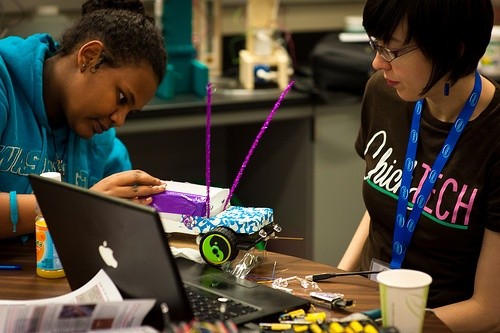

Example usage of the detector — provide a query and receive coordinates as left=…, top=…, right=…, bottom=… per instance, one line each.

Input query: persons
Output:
left=337, top=0, right=500, bottom=333
left=0, top=8, right=168, bottom=237
left=82, top=0, right=145, bottom=19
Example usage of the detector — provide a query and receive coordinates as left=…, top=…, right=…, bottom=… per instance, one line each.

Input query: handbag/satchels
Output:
left=311, top=33, right=377, bottom=96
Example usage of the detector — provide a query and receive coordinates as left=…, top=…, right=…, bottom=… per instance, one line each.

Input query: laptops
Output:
left=28, top=173, right=312, bottom=327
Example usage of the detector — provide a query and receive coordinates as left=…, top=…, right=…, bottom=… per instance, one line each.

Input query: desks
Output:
left=120, top=87, right=320, bottom=139
left=0, top=240, right=453, bottom=333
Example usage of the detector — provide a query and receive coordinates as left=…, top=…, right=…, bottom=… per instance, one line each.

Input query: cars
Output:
left=139, top=180, right=282, bottom=267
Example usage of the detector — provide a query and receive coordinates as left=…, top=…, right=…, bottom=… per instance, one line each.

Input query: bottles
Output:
left=35, top=172, right=66, bottom=279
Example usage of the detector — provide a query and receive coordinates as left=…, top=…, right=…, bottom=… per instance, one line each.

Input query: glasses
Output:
left=368, top=36, right=419, bottom=63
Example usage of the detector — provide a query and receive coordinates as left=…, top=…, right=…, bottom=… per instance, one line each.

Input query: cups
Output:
left=478, top=25, right=500, bottom=78
left=377, top=269, right=433, bottom=333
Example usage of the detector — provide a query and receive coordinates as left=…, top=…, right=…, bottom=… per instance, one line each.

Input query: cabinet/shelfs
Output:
left=213, top=90, right=368, bottom=269
